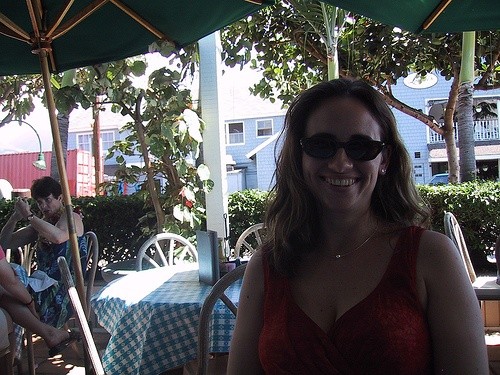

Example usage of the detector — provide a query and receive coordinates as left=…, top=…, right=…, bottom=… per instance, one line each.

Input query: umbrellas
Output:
left=0, top=0, right=500, bottom=319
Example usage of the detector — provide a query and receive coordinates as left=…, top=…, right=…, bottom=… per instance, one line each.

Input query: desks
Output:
left=90, top=261, right=245, bottom=375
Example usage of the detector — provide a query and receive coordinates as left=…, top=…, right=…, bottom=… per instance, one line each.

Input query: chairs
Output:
left=443, top=211, right=500, bottom=310
left=0, top=212, right=269, bottom=375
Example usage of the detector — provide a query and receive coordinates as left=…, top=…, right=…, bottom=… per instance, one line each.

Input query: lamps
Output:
left=0, top=120, right=47, bottom=170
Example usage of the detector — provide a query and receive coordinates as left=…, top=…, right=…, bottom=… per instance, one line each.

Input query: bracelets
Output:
left=25, top=295, right=34, bottom=305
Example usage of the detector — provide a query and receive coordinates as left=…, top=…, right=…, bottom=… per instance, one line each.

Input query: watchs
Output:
left=27, top=213, right=35, bottom=222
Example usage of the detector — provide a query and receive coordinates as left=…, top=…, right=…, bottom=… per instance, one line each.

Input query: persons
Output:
left=0, top=245, right=80, bottom=375
left=227, top=77, right=489, bottom=375
left=0, top=176, right=87, bottom=328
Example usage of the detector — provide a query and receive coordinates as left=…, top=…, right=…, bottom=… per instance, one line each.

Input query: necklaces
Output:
left=319, top=224, right=378, bottom=260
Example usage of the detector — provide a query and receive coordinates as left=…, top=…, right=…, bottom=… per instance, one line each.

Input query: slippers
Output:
left=48, top=330, right=82, bottom=358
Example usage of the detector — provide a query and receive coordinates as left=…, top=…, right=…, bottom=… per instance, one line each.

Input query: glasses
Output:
left=297, top=132, right=387, bottom=163
